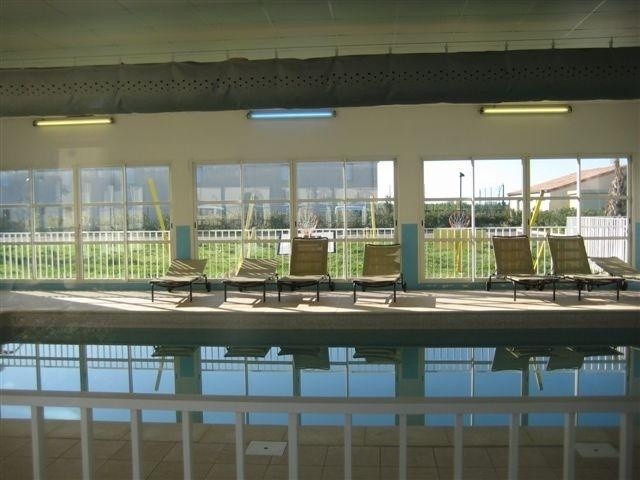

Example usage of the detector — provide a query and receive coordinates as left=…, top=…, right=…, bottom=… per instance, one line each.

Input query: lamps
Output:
left=246, top=111, right=337, bottom=119
left=32, top=118, right=115, bottom=126
left=480, top=107, right=572, bottom=114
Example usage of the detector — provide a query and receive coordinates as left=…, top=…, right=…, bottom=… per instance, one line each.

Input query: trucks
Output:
left=333, top=206, right=372, bottom=226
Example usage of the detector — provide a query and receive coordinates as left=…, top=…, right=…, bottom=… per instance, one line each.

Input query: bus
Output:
left=198, top=206, right=226, bottom=224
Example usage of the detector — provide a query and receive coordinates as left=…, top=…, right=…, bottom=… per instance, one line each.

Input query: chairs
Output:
left=150, top=258, right=209, bottom=304
left=485, top=234, right=559, bottom=303
left=276, top=237, right=334, bottom=302
left=352, top=242, right=408, bottom=304
left=222, top=258, right=279, bottom=302
left=587, top=256, right=640, bottom=283
left=544, top=233, right=625, bottom=302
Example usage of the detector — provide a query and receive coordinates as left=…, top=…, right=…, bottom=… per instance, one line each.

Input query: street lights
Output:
left=459, top=171, right=466, bottom=220
